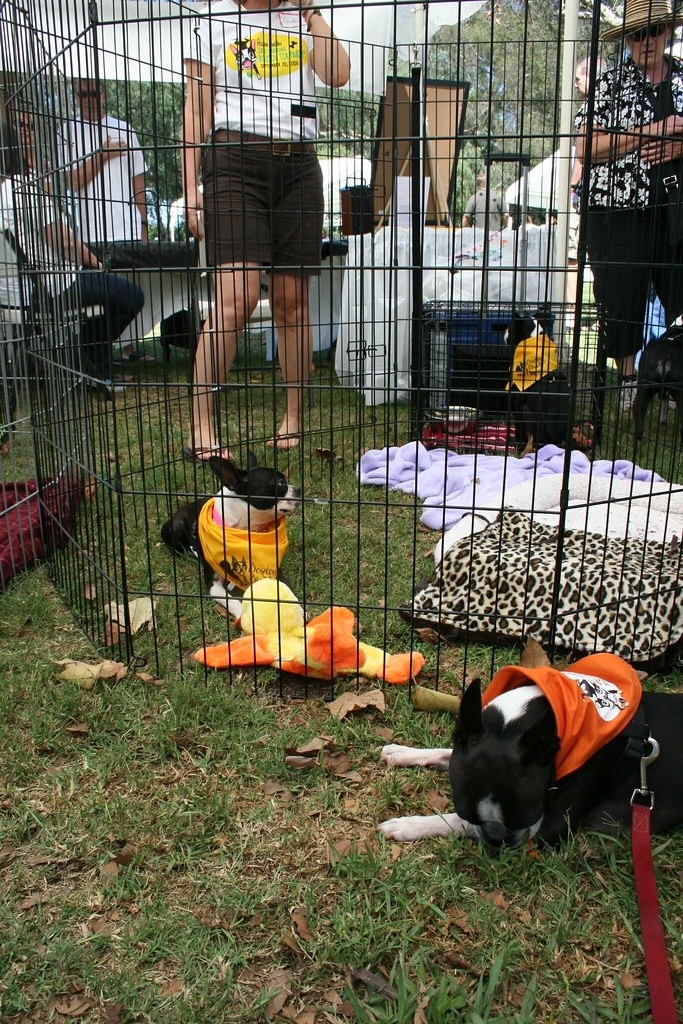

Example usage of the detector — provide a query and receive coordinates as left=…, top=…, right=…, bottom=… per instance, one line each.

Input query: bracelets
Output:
left=307, top=9, right=322, bottom=32
left=571, top=185, right=577, bottom=188
left=142, top=221, right=146, bottom=225
left=302, top=0, right=313, bottom=17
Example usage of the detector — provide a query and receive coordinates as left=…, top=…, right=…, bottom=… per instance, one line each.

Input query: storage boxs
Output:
left=436, top=310, right=555, bottom=382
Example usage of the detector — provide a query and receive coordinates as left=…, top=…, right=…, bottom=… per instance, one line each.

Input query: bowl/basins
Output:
left=425, top=406, right=482, bottom=435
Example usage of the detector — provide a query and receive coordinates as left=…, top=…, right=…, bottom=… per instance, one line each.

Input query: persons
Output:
left=462, top=174, right=509, bottom=231
left=180, top=0, right=352, bottom=464
left=519, top=215, right=536, bottom=230
left=565, top=55, right=607, bottom=334
left=574, top=0, right=683, bottom=411
left=57, top=78, right=155, bottom=362
left=0, top=122, right=145, bottom=363
left=547, top=214, right=557, bottom=225
left=10, top=111, right=50, bottom=173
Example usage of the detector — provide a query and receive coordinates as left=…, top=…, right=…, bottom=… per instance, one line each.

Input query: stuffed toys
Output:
left=195, top=578, right=425, bottom=683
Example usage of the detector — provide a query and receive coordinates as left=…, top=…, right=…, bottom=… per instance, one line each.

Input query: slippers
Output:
left=269, top=429, right=300, bottom=453
left=113, top=359, right=123, bottom=365
left=181, top=444, right=235, bottom=466
left=121, top=350, right=155, bottom=361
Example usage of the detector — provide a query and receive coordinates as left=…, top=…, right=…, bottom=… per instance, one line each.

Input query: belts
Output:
left=650, top=173, right=681, bottom=195
left=212, top=129, right=315, bottom=156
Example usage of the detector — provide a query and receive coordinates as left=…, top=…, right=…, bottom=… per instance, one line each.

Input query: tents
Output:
left=504, top=146, right=576, bottom=229
left=0, top=0, right=577, bottom=347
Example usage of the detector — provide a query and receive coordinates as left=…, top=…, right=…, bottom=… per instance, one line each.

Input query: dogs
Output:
left=375, top=677, right=683, bottom=843
left=503, top=302, right=577, bottom=459
left=160, top=449, right=302, bottom=620
left=632, top=324, right=683, bottom=451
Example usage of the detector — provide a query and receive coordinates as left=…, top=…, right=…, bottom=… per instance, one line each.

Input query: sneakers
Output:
left=669, top=401, right=676, bottom=411
left=618, top=380, right=639, bottom=417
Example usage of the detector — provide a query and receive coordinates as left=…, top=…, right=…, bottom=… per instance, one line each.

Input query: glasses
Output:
left=74, top=89, right=98, bottom=98
left=626, top=23, right=669, bottom=42
left=13, top=121, right=34, bottom=130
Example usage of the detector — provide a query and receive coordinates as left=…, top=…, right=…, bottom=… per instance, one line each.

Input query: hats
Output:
left=600, top=0, right=683, bottom=42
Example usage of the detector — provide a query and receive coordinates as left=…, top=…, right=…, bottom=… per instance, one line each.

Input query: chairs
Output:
left=0, top=228, right=105, bottom=454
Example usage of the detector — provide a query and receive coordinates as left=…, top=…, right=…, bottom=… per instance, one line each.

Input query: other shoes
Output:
left=85, top=378, right=127, bottom=391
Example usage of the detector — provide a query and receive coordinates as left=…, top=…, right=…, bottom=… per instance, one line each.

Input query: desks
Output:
left=84, top=237, right=349, bottom=372
